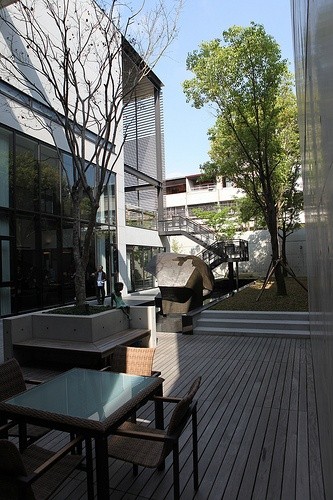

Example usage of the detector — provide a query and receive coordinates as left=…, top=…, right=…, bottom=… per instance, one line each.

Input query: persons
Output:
left=111, top=282, right=133, bottom=320
left=91, top=265, right=107, bottom=305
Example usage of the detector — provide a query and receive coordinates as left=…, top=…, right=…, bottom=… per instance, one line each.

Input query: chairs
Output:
left=110, top=375, right=202, bottom=500
left=98, top=345, right=158, bottom=424
left=0, top=358, right=76, bottom=457
left=0, top=420, right=95, bottom=500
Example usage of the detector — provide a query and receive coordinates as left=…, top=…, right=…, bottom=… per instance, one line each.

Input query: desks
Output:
left=0, top=368, right=166, bottom=500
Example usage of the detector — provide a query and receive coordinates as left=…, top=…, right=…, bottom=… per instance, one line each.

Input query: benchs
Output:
left=15, top=328, right=150, bottom=367
